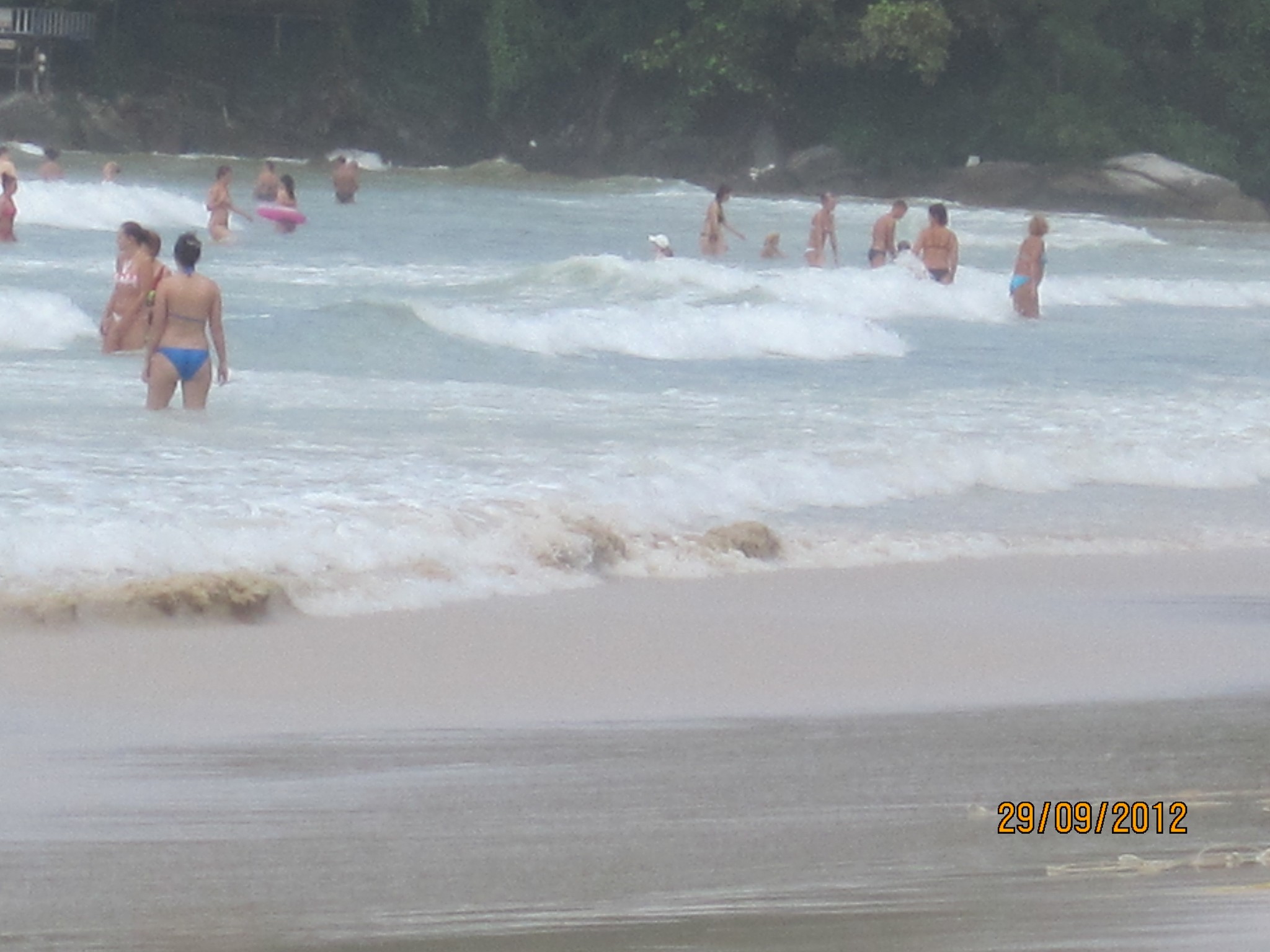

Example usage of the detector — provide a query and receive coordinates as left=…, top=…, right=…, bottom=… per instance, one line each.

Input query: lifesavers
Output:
left=255, top=204, right=306, bottom=224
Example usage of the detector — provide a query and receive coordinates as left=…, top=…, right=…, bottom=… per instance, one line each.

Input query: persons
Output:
left=333, top=156, right=358, bottom=202
left=898, top=239, right=912, bottom=251
left=276, top=174, right=297, bottom=233
left=805, top=191, right=841, bottom=267
left=1012, top=214, right=1047, bottom=317
left=699, top=188, right=746, bottom=255
left=648, top=234, right=673, bottom=257
left=39, top=150, right=64, bottom=180
left=0, top=146, right=18, bottom=180
left=207, top=166, right=252, bottom=243
left=253, top=161, right=279, bottom=201
left=761, top=233, right=785, bottom=259
left=912, top=204, right=959, bottom=286
left=0, top=172, right=19, bottom=242
left=103, top=162, right=119, bottom=182
left=869, top=201, right=907, bottom=266
left=99, top=222, right=172, bottom=353
left=141, top=233, right=228, bottom=410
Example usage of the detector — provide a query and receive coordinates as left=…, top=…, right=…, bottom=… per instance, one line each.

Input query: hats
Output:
left=648, top=234, right=669, bottom=249
